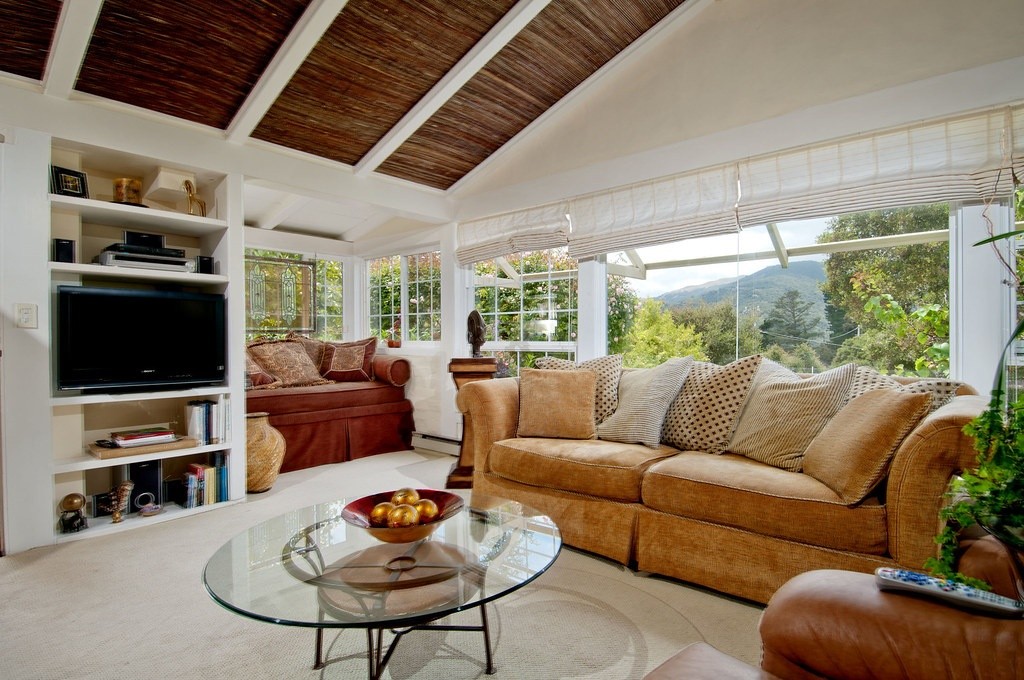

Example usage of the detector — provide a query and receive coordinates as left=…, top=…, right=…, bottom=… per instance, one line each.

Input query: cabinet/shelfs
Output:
left=50, top=138, right=246, bottom=545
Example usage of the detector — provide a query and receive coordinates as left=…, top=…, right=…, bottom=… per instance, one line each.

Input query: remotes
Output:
left=874, top=566, right=1024, bottom=620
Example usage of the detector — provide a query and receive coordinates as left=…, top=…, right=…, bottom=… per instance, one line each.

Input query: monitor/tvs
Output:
left=53, top=284, right=228, bottom=394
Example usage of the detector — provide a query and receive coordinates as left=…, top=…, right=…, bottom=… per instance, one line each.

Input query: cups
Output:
left=388, top=339, right=401, bottom=348
left=113, top=178, right=142, bottom=205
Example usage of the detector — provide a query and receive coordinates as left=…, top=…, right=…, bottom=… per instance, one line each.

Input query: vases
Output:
left=246, top=412, right=286, bottom=494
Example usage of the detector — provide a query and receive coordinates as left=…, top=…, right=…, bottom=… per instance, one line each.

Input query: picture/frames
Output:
left=52, top=165, right=89, bottom=199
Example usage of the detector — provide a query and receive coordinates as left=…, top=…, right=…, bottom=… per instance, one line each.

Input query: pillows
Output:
left=517, top=355, right=965, bottom=507
left=248, top=334, right=378, bottom=387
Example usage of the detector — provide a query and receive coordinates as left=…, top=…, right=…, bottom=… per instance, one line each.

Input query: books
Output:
left=178, top=450, right=229, bottom=509
left=184, top=394, right=232, bottom=445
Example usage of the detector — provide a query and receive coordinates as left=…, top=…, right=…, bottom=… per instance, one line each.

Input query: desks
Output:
left=203, top=494, right=565, bottom=680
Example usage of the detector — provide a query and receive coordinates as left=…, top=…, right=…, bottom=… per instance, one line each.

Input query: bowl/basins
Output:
left=342, top=489, right=465, bottom=544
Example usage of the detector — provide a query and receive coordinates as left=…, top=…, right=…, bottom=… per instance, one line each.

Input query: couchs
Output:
left=644, top=569, right=1024, bottom=680
left=244, top=334, right=416, bottom=472
left=457, top=361, right=997, bottom=607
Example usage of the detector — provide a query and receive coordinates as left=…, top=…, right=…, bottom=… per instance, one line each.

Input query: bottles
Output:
left=246, top=412, right=286, bottom=494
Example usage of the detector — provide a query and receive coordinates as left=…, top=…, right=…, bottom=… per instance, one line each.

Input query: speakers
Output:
left=194, top=255, right=215, bottom=274
left=111, top=458, right=165, bottom=515
left=51, top=238, right=76, bottom=263
